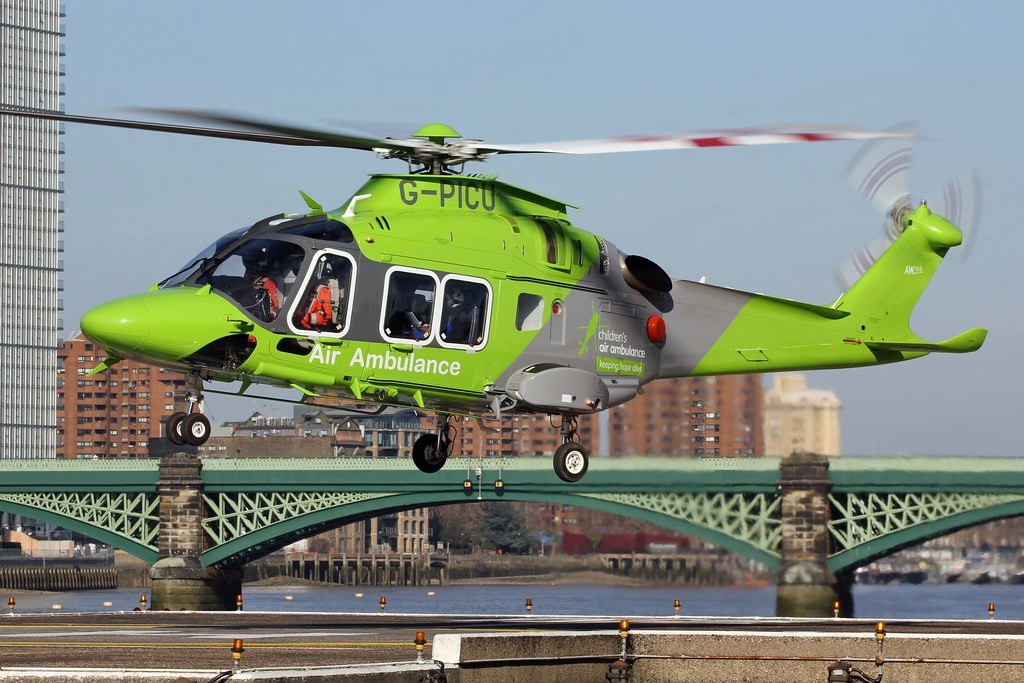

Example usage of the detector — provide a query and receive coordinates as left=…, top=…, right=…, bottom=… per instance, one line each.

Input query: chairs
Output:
left=392, top=294, right=433, bottom=324
left=320, top=263, right=339, bottom=325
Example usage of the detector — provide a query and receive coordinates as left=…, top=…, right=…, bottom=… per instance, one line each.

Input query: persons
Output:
left=408, top=286, right=471, bottom=343
left=242, top=247, right=332, bottom=330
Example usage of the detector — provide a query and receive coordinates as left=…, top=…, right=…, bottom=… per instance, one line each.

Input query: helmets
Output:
left=293, top=253, right=332, bottom=276
left=246, top=247, right=281, bottom=276
left=445, top=286, right=465, bottom=308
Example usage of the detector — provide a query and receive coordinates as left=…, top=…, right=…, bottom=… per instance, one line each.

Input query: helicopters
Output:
left=0, top=83, right=988, bottom=496
